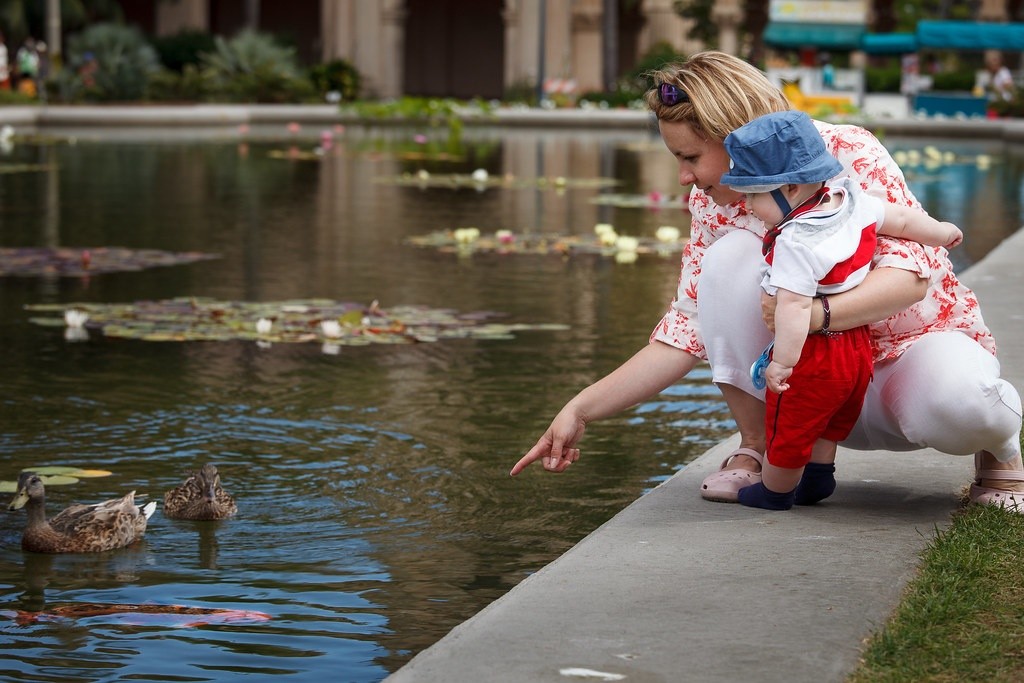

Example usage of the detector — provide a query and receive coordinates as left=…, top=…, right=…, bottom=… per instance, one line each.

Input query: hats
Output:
left=719, top=110, right=844, bottom=194
left=728, top=184, right=785, bottom=194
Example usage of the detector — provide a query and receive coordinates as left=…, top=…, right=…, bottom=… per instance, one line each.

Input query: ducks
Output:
left=6, top=471, right=158, bottom=554
left=163, top=464, right=239, bottom=520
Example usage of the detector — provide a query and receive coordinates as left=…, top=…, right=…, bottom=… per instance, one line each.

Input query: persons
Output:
left=509, top=50, right=1024, bottom=516
left=720, top=110, right=963, bottom=510
left=975, top=50, right=1013, bottom=100
left=899, top=51, right=931, bottom=112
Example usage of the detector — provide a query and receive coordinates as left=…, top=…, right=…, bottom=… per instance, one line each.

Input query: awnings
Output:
left=762, top=23, right=862, bottom=50
left=917, top=19, right=1024, bottom=51
left=862, top=34, right=917, bottom=51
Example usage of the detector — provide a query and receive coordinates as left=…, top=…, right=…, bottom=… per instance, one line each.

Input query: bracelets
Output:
left=817, top=296, right=842, bottom=335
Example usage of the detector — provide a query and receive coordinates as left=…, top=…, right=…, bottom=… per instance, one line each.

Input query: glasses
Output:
left=646, top=77, right=690, bottom=107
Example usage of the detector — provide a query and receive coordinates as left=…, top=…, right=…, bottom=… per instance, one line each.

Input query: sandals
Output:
left=700, top=448, right=764, bottom=503
left=970, top=450, right=1024, bottom=515
